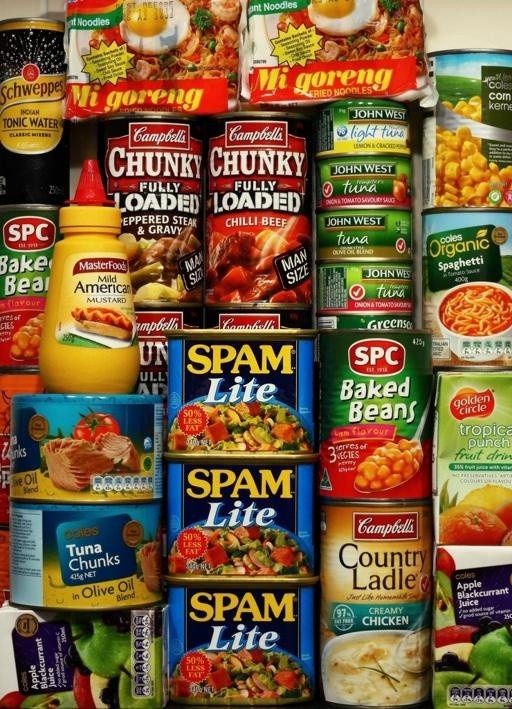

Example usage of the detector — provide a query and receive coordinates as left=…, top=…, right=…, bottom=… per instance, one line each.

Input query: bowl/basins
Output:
left=321, top=630, right=430, bottom=706
left=435, top=280, right=511, bottom=362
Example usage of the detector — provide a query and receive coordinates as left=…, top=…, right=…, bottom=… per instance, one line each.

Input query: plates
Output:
left=68, top=320, right=137, bottom=349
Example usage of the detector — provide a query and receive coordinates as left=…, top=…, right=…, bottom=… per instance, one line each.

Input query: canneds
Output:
left=0, top=18, right=512, bottom=709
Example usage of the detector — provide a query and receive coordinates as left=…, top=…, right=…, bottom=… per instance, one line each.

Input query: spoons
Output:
left=393, top=627, right=430, bottom=673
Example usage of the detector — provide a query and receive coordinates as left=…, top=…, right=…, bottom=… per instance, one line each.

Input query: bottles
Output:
left=40, top=155, right=146, bottom=395
